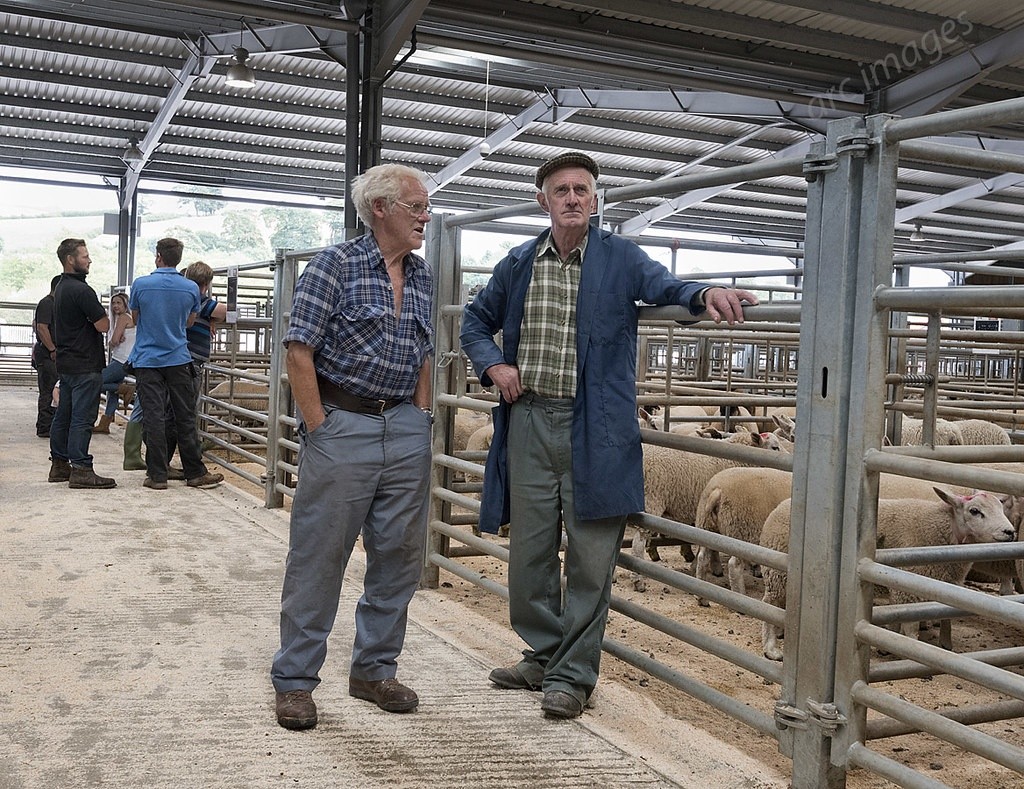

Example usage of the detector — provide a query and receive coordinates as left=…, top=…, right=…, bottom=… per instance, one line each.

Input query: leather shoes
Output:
left=349, top=676, right=419, bottom=711
left=275, top=690, right=317, bottom=728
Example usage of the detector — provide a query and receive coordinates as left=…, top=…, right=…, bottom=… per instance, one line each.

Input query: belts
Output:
left=317, top=376, right=407, bottom=414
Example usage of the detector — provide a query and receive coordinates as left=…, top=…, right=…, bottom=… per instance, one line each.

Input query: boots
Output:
left=93, top=415, right=113, bottom=434
left=114, top=384, right=135, bottom=407
left=123, top=422, right=147, bottom=470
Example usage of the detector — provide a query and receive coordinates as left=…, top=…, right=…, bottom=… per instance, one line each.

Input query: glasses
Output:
left=395, top=201, right=432, bottom=217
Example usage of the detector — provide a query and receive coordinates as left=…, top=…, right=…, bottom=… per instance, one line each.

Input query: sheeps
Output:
left=206, top=380, right=269, bottom=444
left=450, top=392, right=1024, bottom=616
left=759, top=485, right=1016, bottom=663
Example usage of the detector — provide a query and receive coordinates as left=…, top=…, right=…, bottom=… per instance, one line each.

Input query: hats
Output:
left=535, top=152, right=599, bottom=190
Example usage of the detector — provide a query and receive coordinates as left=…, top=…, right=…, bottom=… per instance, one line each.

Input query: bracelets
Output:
left=421, top=407, right=434, bottom=418
left=50, top=348, right=56, bottom=352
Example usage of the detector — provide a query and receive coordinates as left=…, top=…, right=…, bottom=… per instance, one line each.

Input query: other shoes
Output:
left=68, top=462, right=116, bottom=488
left=48, top=459, right=73, bottom=482
left=488, top=668, right=529, bottom=687
left=541, top=690, right=582, bottom=718
left=38, top=431, right=50, bottom=437
left=143, top=478, right=167, bottom=489
left=187, top=472, right=224, bottom=488
left=167, top=466, right=185, bottom=480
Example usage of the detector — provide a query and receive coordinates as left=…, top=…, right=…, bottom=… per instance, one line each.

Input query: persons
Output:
left=30, top=239, right=226, bottom=489
left=460, top=152, right=760, bottom=714
left=271, top=164, right=434, bottom=730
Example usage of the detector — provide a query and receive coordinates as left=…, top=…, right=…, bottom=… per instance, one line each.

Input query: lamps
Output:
left=224, top=14, right=271, bottom=90
left=908, top=215, right=927, bottom=242
left=121, top=120, right=145, bottom=163
